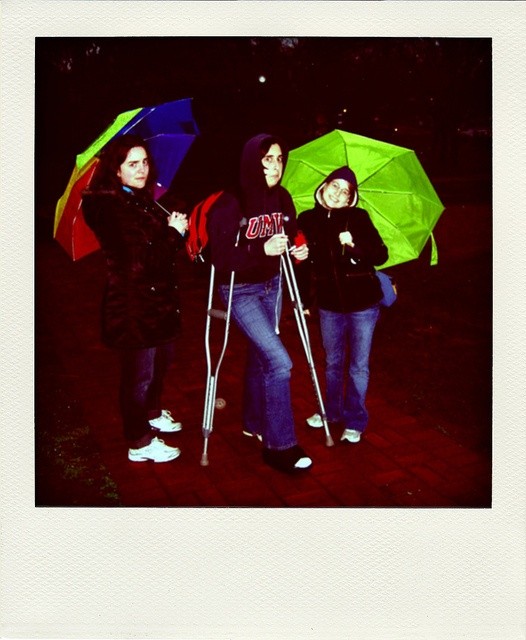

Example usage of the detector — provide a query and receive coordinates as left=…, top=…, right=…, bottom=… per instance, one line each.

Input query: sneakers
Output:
left=306, top=413, right=324, bottom=428
left=127, top=437, right=181, bottom=463
left=148, top=409, right=182, bottom=432
left=340, top=428, right=362, bottom=443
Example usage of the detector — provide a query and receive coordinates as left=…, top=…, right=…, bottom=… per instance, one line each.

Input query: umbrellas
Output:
left=279, top=129, right=445, bottom=271
left=52, top=98, right=201, bottom=263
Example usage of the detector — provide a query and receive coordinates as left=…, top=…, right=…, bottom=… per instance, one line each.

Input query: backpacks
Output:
left=183, top=189, right=247, bottom=276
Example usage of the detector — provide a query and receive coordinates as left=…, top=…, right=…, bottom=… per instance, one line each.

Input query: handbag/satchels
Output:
left=336, top=209, right=375, bottom=283
left=374, top=270, right=397, bottom=307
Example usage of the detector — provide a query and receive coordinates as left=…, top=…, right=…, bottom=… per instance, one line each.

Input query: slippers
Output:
left=262, top=445, right=312, bottom=474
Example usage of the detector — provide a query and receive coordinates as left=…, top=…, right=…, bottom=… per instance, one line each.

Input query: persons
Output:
left=295, top=165, right=388, bottom=445
left=80, top=136, right=190, bottom=465
left=206, top=133, right=312, bottom=477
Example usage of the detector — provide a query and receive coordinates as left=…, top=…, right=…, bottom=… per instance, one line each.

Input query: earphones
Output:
left=275, top=325, right=280, bottom=335
left=289, top=245, right=296, bottom=251
